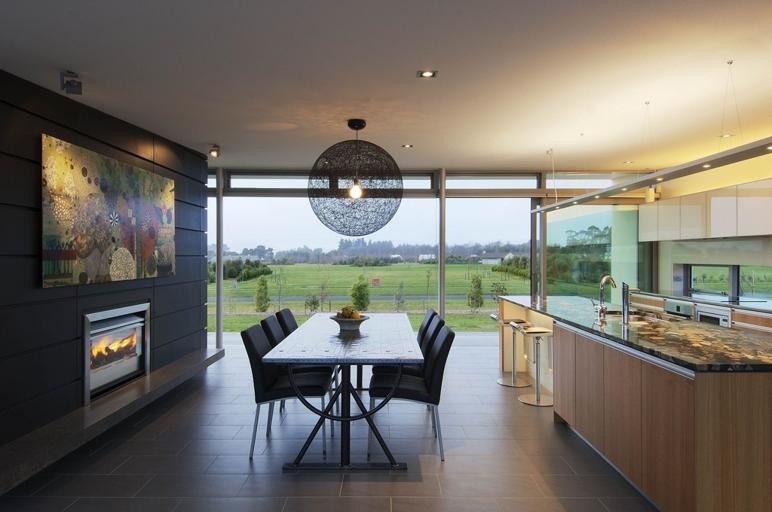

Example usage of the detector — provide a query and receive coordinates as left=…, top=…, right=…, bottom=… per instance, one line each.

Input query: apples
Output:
left=342, top=307, right=353, bottom=318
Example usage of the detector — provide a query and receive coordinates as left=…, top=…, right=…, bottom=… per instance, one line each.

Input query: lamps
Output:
left=304, top=118, right=411, bottom=240
left=56, top=68, right=85, bottom=97
left=207, top=140, right=223, bottom=159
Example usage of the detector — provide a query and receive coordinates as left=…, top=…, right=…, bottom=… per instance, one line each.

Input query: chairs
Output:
left=365, top=302, right=438, bottom=411
left=235, top=323, right=332, bottom=463
left=272, top=305, right=342, bottom=417
left=364, top=322, right=456, bottom=462
left=257, top=314, right=336, bottom=437
left=369, top=313, right=447, bottom=427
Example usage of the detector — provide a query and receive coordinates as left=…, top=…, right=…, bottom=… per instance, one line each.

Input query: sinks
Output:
left=604, top=309, right=647, bottom=325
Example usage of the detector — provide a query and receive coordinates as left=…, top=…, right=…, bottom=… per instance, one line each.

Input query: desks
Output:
left=262, top=308, right=426, bottom=473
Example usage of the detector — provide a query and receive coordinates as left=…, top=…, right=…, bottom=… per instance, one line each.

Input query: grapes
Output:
left=337, top=312, right=342, bottom=318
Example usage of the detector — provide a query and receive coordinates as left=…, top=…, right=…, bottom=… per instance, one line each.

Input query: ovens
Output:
left=664, top=299, right=695, bottom=318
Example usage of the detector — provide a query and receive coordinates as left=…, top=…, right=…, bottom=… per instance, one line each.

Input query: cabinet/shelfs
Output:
left=681, top=186, right=738, bottom=238
left=602, top=346, right=692, bottom=511
left=738, top=176, right=772, bottom=237
left=552, top=327, right=604, bottom=456
left=636, top=195, right=681, bottom=243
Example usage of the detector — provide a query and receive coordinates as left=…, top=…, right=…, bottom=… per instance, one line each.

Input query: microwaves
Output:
left=694, top=303, right=731, bottom=328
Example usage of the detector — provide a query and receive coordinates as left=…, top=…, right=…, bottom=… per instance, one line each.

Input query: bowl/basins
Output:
left=329, top=314, right=369, bottom=331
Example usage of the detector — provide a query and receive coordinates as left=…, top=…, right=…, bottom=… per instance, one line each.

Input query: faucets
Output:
left=621, top=282, right=641, bottom=323
left=595, top=273, right=616, bottom=326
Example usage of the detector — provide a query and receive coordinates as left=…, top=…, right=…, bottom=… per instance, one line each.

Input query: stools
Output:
left=489, top=310, right=535, bottom=391
left=507, top=320, right=557, bottom=408
